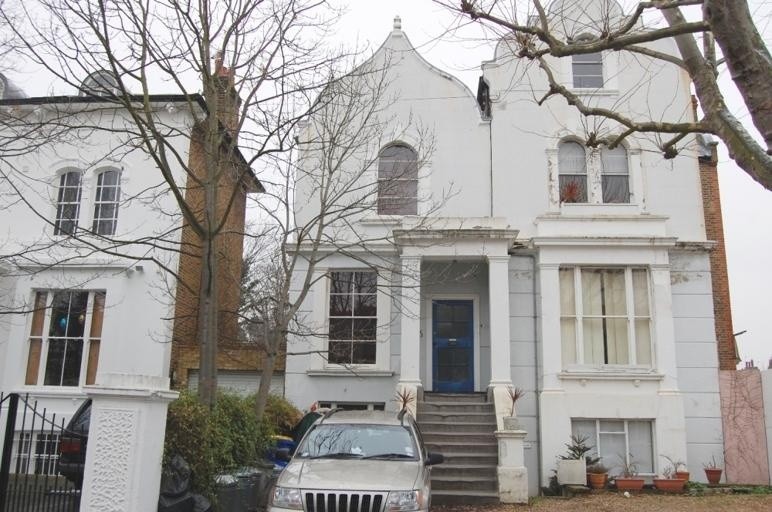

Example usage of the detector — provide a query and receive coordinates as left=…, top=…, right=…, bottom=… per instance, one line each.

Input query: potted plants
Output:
left=613, top=450, right=645, bottom=489
left=702, top=452, right=722, bottom=483
left=589, top=462, right=608, bottom=490
left=550, top=431, right=590, bottom=489
left=652, top=467, right=685, bottom=490
left=672, top=462, right=691, bottom=481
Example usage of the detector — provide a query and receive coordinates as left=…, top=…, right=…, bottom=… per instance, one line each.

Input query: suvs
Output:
left=58, top=397, right=92, bottom=490
left=268, top=409, right=443, bottom=512
